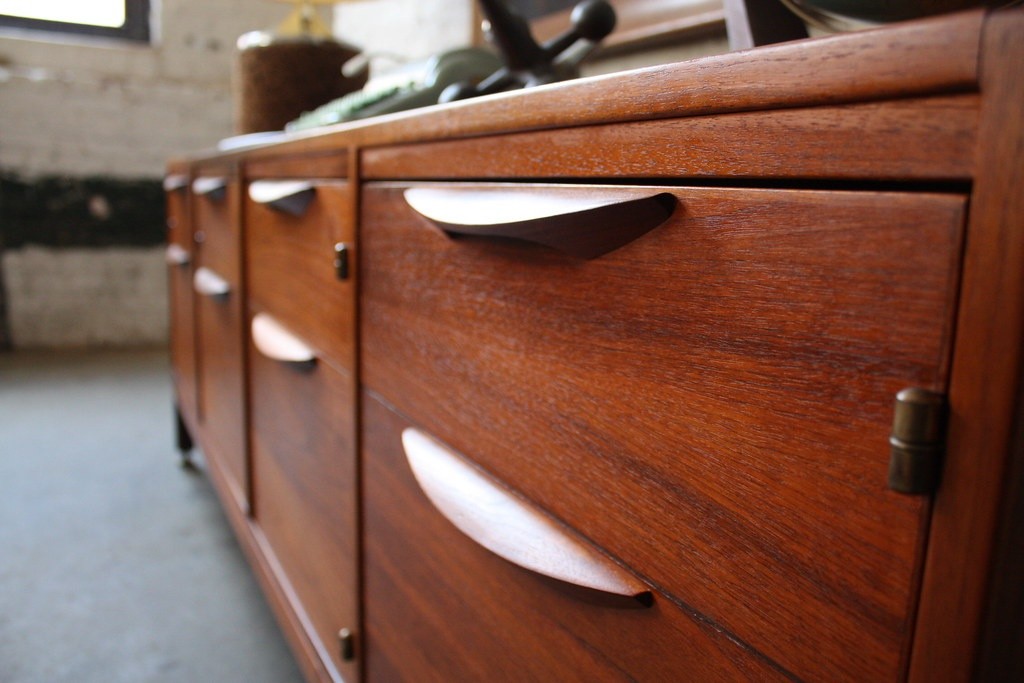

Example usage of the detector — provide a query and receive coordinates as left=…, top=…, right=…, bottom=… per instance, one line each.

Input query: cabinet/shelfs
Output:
left=164, top=9, right=1024, bottom=683
